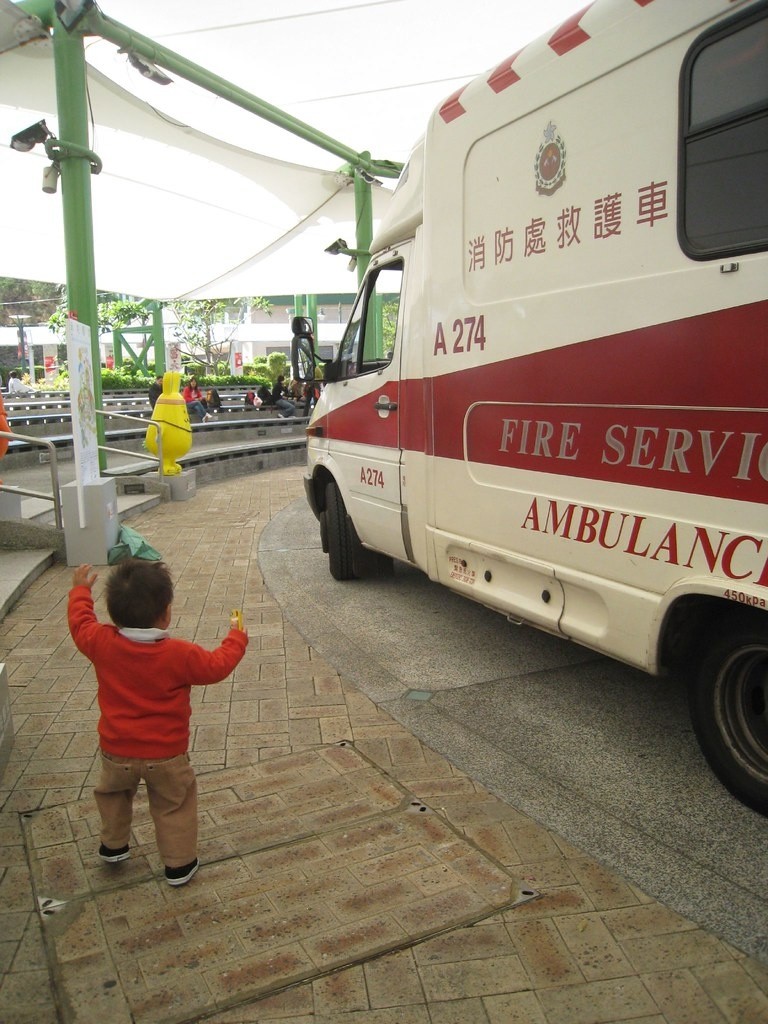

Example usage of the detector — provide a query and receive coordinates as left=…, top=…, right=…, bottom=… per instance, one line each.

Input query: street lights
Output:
left=134, top=309, right=153, bottom=372
left=8, top=310, right=32, bottom=377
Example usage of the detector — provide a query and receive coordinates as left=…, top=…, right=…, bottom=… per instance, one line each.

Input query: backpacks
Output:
left=245, top=386, right=274, bottom=406
left=206, top=388, right=222, bottom=407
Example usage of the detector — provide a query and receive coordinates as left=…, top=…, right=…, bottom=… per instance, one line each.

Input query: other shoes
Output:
left=289, top=416, right=295, bottom=418
left=165, top=857, right=200, bottom=885
left=99, top=843, right=130, bottom=862
left=202, top=415, right=208, bottom=421
left=205, top=413, right=212, bottom=418
left=278, top=413, right=284, bottom=418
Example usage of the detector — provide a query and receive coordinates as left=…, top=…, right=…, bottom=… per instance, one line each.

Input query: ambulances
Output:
left=290, top=0, right=768, bottom=822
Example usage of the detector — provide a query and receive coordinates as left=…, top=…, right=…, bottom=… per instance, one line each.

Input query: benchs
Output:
left=0, top=385, right=310, bottom=451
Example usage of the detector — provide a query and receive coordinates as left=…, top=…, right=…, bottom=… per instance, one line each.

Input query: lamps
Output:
left=129, top=52, right=174, bottom=86
left=324, top=238, right=348, bottom=256
left=9, top=120, right=52, bottom=153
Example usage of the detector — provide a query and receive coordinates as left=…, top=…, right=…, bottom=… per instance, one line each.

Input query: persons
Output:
left=8, top=371, right=40, bottom=392
left=289, top=359, right=323, bottom=410
left=183, top=377, right=212, bottom=423
left=148, top=375, right=163, bottom=409
left=68, top=558, right=249, bottom=886
left=209, top=389, right=222, bottom=407
left=271, top=375, right=296, bottom=418
